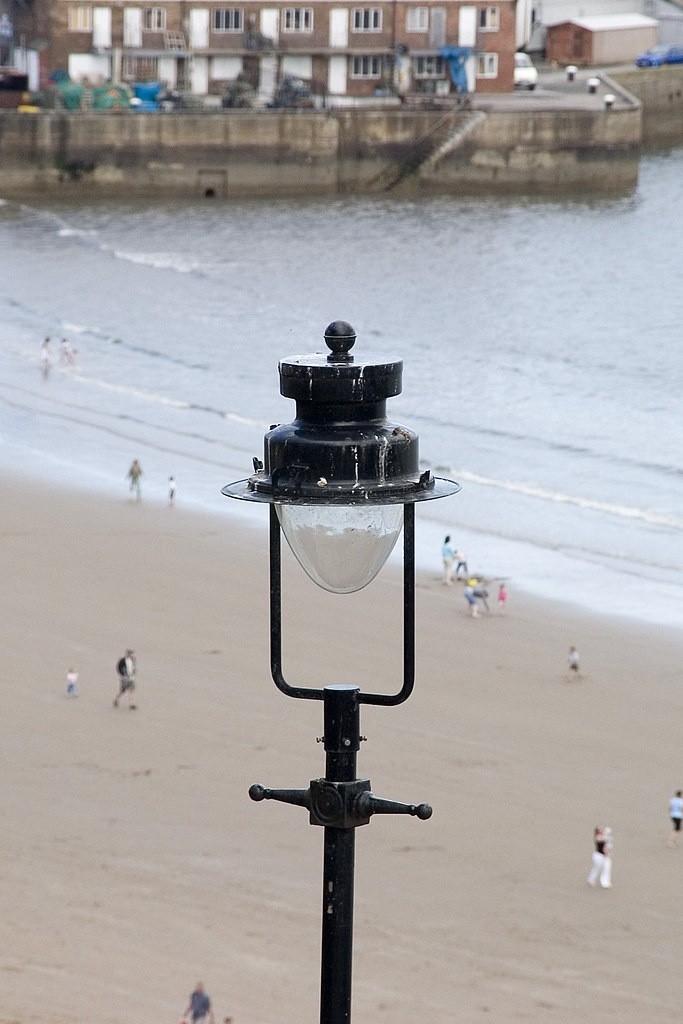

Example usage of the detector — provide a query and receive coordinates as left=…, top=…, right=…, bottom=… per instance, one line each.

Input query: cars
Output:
left=636, top=42, right=683, bottom=67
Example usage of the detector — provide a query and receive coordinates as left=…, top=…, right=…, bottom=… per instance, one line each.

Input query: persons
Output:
left=568, top=647, right=584, bottom=678
left=587, top=825, right=613, bottom=889
left=498, top=585, right=508, bottom=613
left=667, top=791, right=682, bottom=831
left=169, top=474, right=176, bottom=504
left=442, top=536, right=468, bottom=585
left=128, top=458, right=142, bottom=490
left=66, top=668, right=78, bottom=695
left=113, top=648, right=137, bottom=709
left=464, top=579, right=480, bottom=618
left=183, top=981, right=213, bottom=1024
left=41, top=336, right=72, bottom=366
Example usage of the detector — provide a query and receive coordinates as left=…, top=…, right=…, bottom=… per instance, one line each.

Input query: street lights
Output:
left=221, top=321, right=463, bottom=1023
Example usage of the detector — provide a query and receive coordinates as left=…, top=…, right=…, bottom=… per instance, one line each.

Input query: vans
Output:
left=513, top=52, right=539, bottom=91
